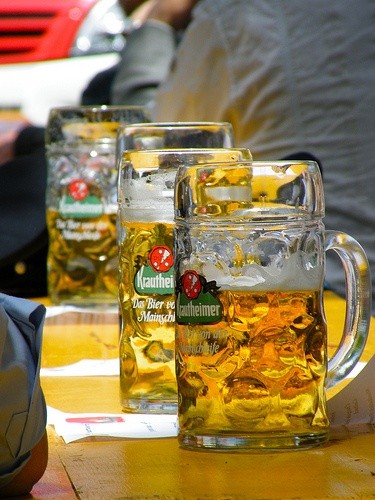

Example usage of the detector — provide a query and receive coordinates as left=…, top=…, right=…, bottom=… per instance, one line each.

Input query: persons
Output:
left=0, top=294, right=49, bottom=500
left=110, top=0, right=375, bottom=314
left=0, top=68, right=114, bottom=298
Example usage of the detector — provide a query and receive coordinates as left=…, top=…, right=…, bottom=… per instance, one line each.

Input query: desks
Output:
left=24, top=289, right=375, bottom=500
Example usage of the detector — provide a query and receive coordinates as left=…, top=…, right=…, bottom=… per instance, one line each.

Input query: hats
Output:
left=0, top=157, right=50, bottom=297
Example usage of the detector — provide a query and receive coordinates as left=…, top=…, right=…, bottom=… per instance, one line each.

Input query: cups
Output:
left=172, top=158, right=372, bottom=454
left=116, top=124, right=237, bottom=156
left=115, top=148, right=258, bottom=416
left=44, top=103, right=156, bottom=307
